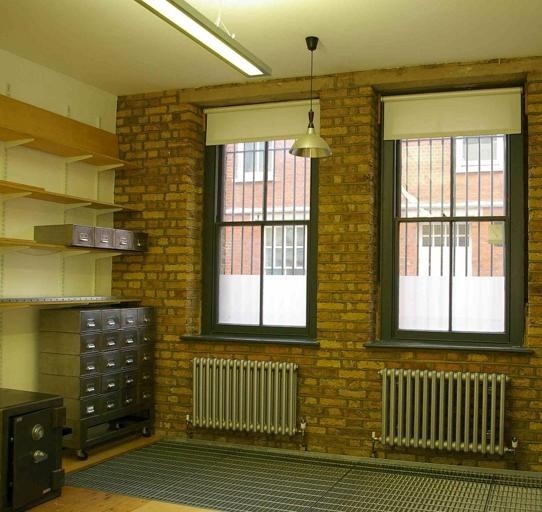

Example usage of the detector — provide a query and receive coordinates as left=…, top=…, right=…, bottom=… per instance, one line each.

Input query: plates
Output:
left=33, top=224, right=150, bottom=252
left=38, top=306, right=155, bottom=459
left=2, top=90, right=143, bottom=307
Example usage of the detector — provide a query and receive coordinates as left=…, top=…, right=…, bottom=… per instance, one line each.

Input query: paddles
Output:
left=61, top=435, right=542, bottom=511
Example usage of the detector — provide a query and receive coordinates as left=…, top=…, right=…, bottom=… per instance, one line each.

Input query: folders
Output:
left=369, top=367, right=520, bottom=470
left=184, top=354, right=308, bottom=450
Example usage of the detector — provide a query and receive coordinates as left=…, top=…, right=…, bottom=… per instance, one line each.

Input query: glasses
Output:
left=288, top=36, right=334, bottom=160
left=136, top=0, right=273, bottom=80
